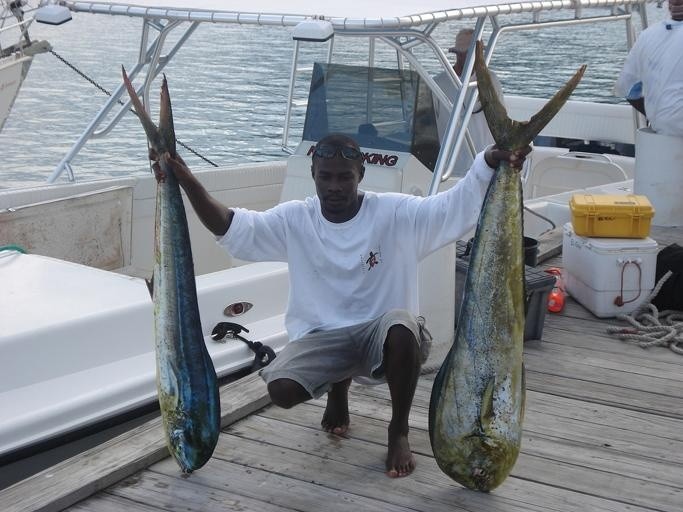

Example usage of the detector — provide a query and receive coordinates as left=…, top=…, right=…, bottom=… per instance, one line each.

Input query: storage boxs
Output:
left=562, top=222, right=659, bottom=320
left=455, top=239, right=557, bottom=342
left=569, top=194, right=655, bottom=240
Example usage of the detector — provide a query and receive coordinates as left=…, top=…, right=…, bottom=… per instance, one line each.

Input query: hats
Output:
left=449, top=29, right=483, bottom=55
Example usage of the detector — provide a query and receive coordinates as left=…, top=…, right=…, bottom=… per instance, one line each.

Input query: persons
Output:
left=146, top=132, right=533, bottom=479
left=613, top=1, right=682, bottom=137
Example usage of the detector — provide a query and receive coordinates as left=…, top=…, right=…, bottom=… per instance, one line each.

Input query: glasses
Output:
left=312, top=143, right=364, bottom=164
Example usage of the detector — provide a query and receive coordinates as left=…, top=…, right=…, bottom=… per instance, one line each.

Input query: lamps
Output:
left=291, top=19, right=334, bottom=42
left=35, top=4, right=73, bottom=25
